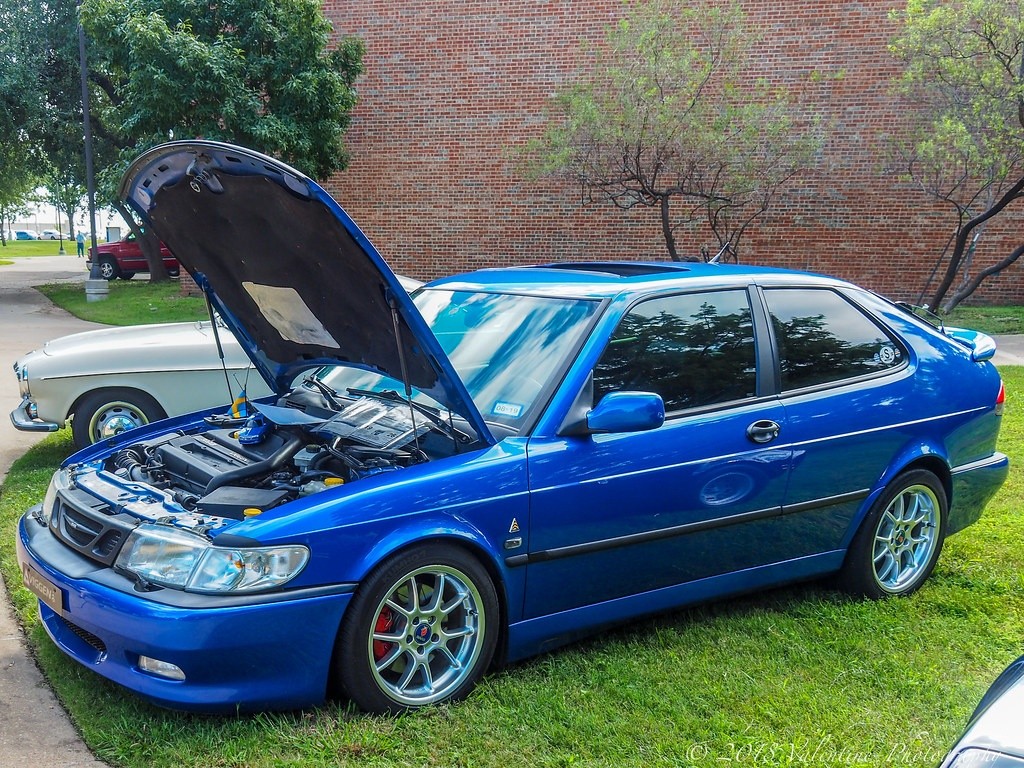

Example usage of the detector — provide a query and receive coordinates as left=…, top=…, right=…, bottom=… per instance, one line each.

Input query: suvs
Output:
left=85, top=223, right=180, bottom=279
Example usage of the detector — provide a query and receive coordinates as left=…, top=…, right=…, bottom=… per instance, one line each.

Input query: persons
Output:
left=76, top=230, right=86, bottom=257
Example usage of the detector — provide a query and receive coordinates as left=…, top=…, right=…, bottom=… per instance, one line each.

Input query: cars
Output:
left=0, top=229, right=17, bottom=241
left=15, top=230, right=38, bottom=240
left=12, top=139, right=1012, bottom=720
left=37, top=229, right=69, bottom=240
left=10, top=273, right=472, bottom=453
